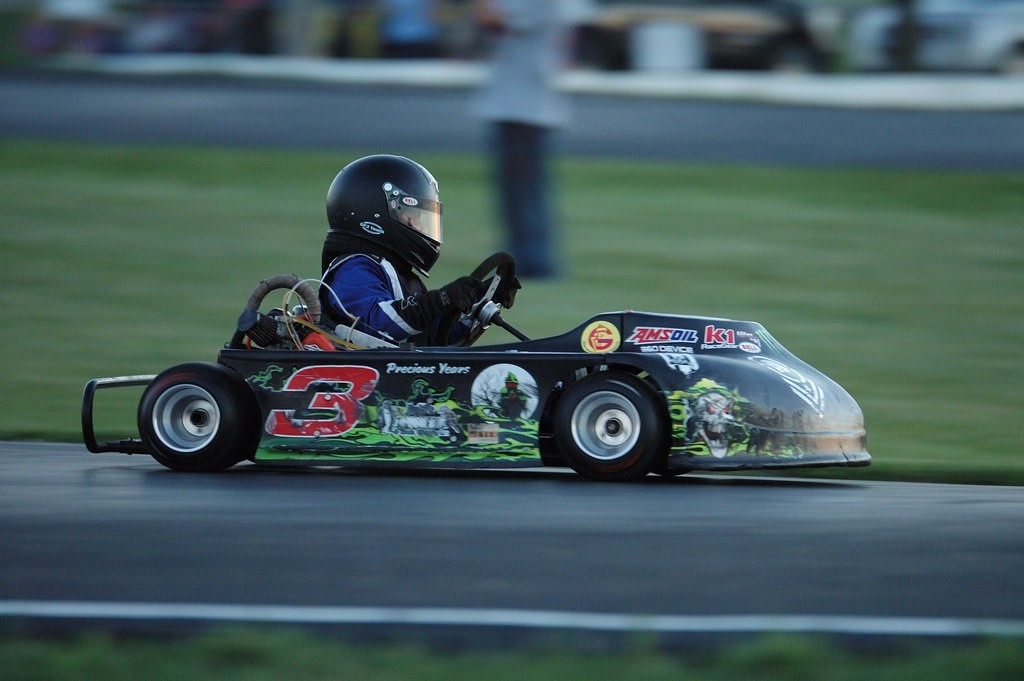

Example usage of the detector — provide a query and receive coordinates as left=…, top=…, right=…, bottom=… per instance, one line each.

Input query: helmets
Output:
left=326, top=154, right=443, bottom=278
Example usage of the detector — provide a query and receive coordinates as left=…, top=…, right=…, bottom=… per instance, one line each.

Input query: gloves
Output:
left=483, top=275, right=522, bottom=309
left=419, top=276, right=485, bottom=324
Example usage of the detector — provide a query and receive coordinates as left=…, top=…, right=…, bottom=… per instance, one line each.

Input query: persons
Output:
left=317, top=155, right=522, bottom=351
left=473, top=0, right=574, bottom=280
